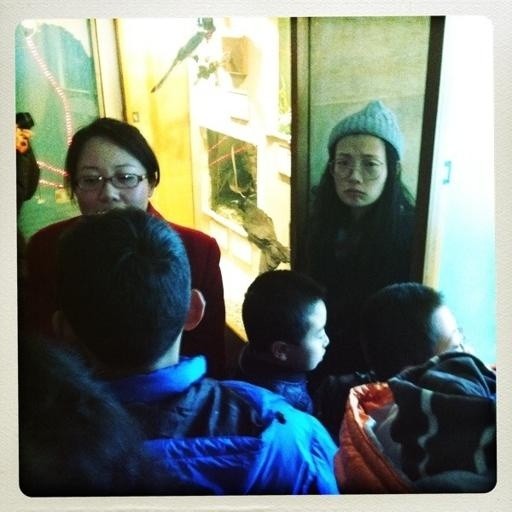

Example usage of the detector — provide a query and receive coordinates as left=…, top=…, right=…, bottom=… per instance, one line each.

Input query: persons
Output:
left=57, top=209, right=338, bottom=494
left=62, top=114, right=228, bottom=380
left=302, top=97, right=415, bottom=336
left=218, top=268, right=376, bottom=447
left=369, top=283, right=495, bottom=386
left=17, top=125, right=42, bottom=255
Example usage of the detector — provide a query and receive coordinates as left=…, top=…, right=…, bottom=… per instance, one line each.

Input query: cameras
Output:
left=16, top=111, right=34, bottom=132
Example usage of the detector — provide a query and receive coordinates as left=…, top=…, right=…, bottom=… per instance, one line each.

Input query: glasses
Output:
left=324, top=160, right=390, bottom=182
left=72, top=173, right=149, bottom=189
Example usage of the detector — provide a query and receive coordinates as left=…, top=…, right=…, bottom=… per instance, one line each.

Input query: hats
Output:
left=327, top=100, right=405, bottom=162
